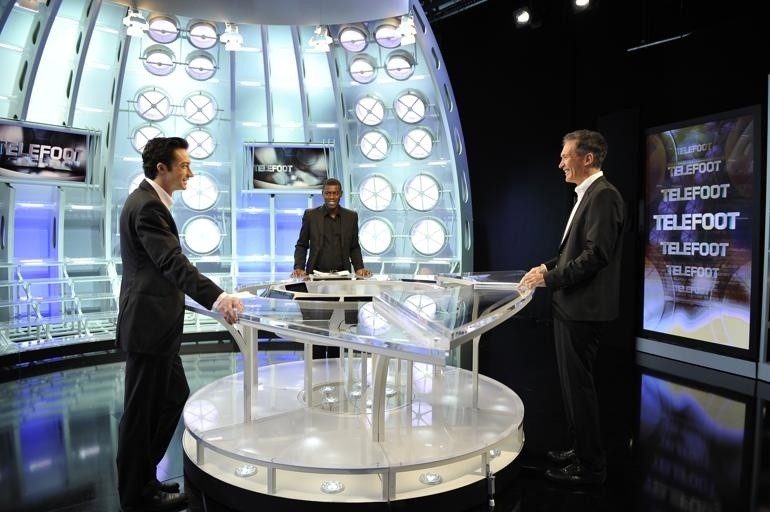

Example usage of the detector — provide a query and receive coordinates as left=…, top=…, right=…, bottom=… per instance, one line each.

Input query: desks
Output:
left=185, top=273, right=536, bottom=442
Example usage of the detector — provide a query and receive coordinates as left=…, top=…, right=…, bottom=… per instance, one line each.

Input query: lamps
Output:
left=398, top=16, right=416, bottom=44
left=309, top=26, right=333, bottom=50
left=122, top=9, right=150, bottom=37
left=220, top=22, right=243, bottom=51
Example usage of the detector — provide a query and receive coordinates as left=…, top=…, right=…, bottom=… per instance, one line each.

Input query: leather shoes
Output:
left=545, top=464, right=607, bottom=488
left=546, top=449, right=577, bottom=464
left=119, top=480, right=185, bottom=511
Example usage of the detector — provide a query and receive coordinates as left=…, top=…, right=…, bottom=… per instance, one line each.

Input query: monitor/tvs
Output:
left=0, top=117, right=102, bottom=191
left=238, top=143, right=338, bottom=197
left=633, top=104, right=765, bottom=364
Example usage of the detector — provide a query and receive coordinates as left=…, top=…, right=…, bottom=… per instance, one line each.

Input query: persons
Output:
left=116, top=136, right=243, bottom=512
left=293, top=178, right=370, bottom=278
left=517, top=129, right=629, bottom=485
